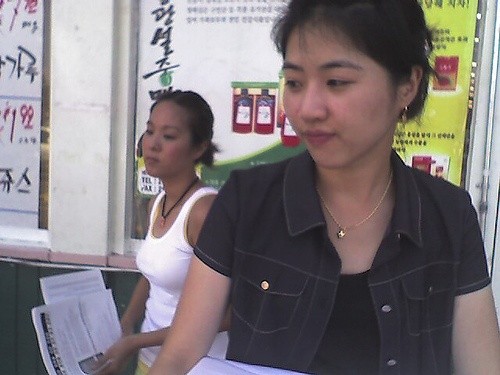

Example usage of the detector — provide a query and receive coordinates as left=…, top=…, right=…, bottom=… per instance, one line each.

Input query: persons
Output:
left=146, top=1, right=500, bottom=375
left=88, top=87, right=232, bottom=375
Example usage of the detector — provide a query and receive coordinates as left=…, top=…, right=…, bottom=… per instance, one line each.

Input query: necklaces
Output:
left=160, top=176, right=199, bottom=225
left=314, top=171, right=395, bottom=241
